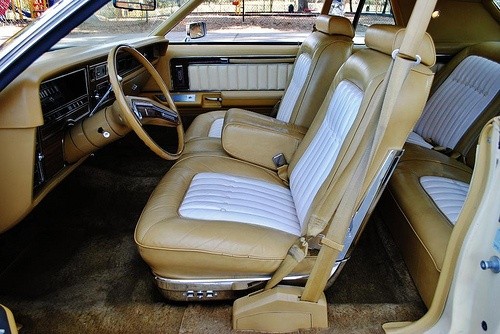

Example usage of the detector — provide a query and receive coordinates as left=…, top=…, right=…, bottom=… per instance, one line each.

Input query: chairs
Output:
left=134, top=25, right=436, bottom=301
left=184, top=15, right=355, bottom=155
left=385, top=41, right=500, bottom=311
